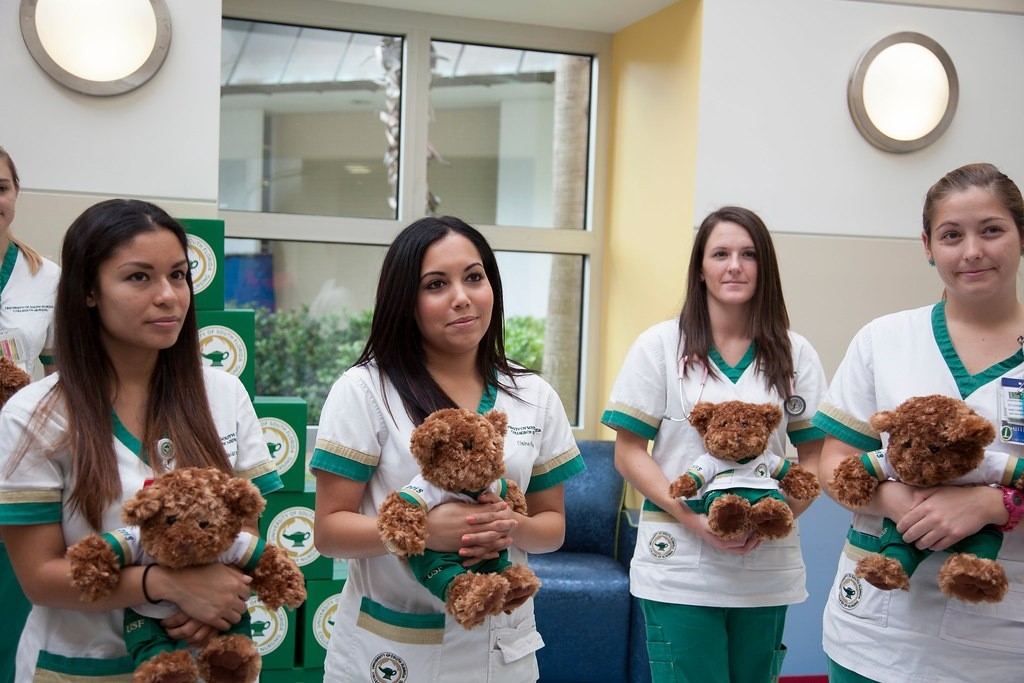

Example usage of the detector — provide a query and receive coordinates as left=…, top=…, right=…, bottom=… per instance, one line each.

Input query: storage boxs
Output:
left=172, top=216, right=346, bottom=683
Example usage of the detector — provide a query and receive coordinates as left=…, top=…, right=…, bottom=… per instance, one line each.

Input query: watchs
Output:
left=989, top=483, right=1024, bottom=533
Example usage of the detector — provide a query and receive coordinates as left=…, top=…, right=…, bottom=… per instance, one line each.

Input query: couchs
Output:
left=528, top=439, right=651, bottom=683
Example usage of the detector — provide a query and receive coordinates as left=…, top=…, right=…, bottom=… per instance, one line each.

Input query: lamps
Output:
left=848, top=31, right=960, bottom=152
left=19, top=0, right=172, bottom=95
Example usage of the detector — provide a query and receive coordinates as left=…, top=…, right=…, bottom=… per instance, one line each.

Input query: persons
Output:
left=810, top=164, right=1024, bottom=683
left=0, top=143, right=284, bottom=683
left=309, top=215, right=587, bottom=683
left=601, top=207, right=826, bottom=683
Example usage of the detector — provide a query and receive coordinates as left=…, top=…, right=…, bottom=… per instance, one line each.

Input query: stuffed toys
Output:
left=669, top=400, right=821, bottom=542
left=828, top=394, right=1024, bottom=604
left=0, top=356, right=31, bottom=411
left=375, top=408, right=542, bottom=629
left=65, top=468, right=307, bottom=683
